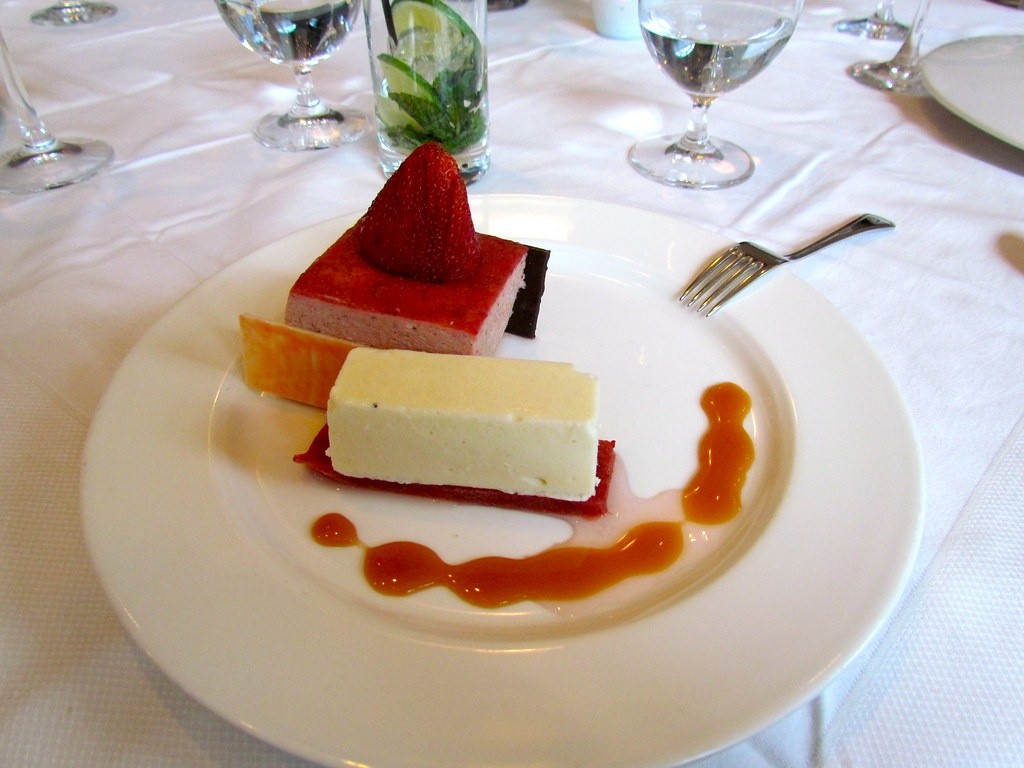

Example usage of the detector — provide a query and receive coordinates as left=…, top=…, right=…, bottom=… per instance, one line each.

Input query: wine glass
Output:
left=29, top=0, right=118, bottom=27
left=832, top=0, right=909, bottom=42
left=213, top=0, right=369, bottom=153
left=847, top=0, right=933, bottom=96
left=0, top=33, right=114, bottom=195
left=627, top=0, right=805, bottom=191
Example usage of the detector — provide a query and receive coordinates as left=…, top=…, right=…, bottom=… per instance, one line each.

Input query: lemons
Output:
left=372, top=0, right=481, bottom=135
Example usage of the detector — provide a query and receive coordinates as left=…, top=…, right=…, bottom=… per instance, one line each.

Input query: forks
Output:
left=678, top=213, right=895, bottom=318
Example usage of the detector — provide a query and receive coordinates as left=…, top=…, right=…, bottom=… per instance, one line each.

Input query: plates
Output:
left=77, top=192, right=926, bottom=766
left=917, top=33, right=1024, bottom=151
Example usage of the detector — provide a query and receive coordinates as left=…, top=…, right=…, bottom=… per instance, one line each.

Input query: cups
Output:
left=362, top=0, right=491, bottom=186
left=590, top=0, right=643, bottom=40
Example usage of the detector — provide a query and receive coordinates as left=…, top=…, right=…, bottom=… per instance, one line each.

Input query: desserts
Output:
left=294, top=345, right=615, bottom=519
left=237, top=141, right=552, bottom=410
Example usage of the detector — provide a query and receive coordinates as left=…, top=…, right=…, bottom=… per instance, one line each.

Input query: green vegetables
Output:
left=387, top=43, right=496, bottom=156
